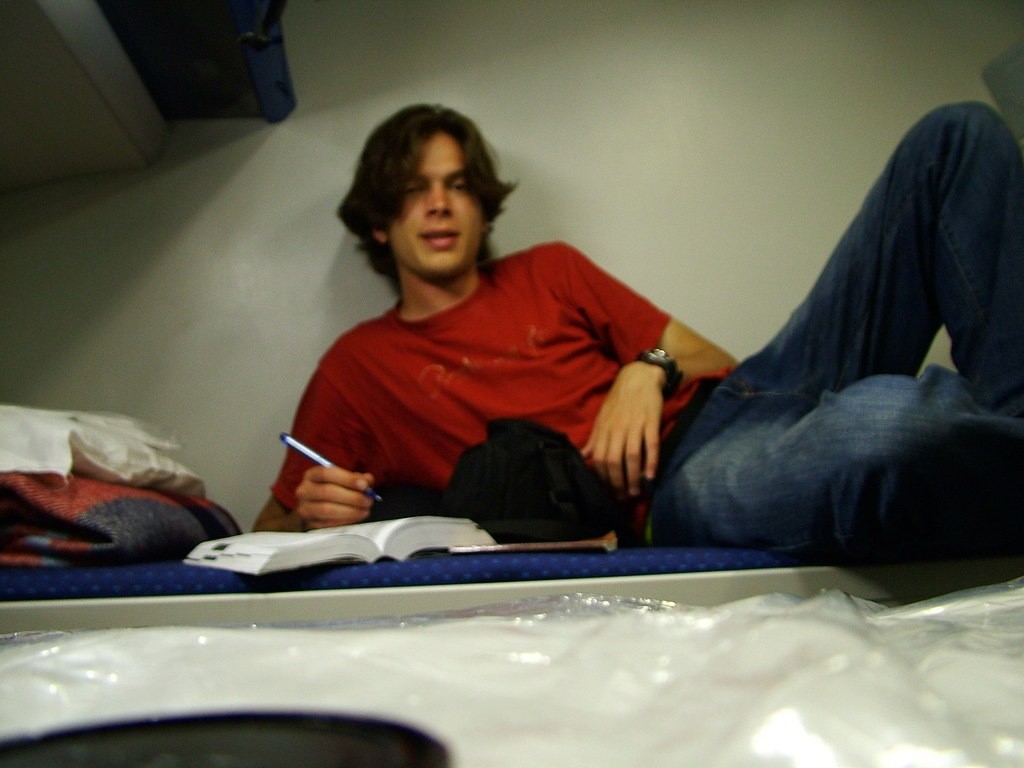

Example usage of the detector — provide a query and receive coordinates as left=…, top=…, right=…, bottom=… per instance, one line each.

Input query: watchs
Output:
left=634, top=347, right=684, bottom=400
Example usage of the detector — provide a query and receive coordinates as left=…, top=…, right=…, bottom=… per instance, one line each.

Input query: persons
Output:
left=252, top=101, right=1024, bottom=544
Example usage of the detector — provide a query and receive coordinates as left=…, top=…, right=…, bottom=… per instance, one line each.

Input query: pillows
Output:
left=0, top=404, right=207, bottom=499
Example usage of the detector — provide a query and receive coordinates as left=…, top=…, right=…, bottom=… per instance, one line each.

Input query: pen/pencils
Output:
left=280, top=432, right=384, bottom=502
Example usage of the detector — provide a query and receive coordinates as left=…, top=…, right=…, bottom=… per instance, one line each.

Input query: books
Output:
left=183, top=517, right=497, bottom=575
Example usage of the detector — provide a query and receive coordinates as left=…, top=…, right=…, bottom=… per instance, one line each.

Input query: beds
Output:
left=0, top=402, right=1024, bottom=768
